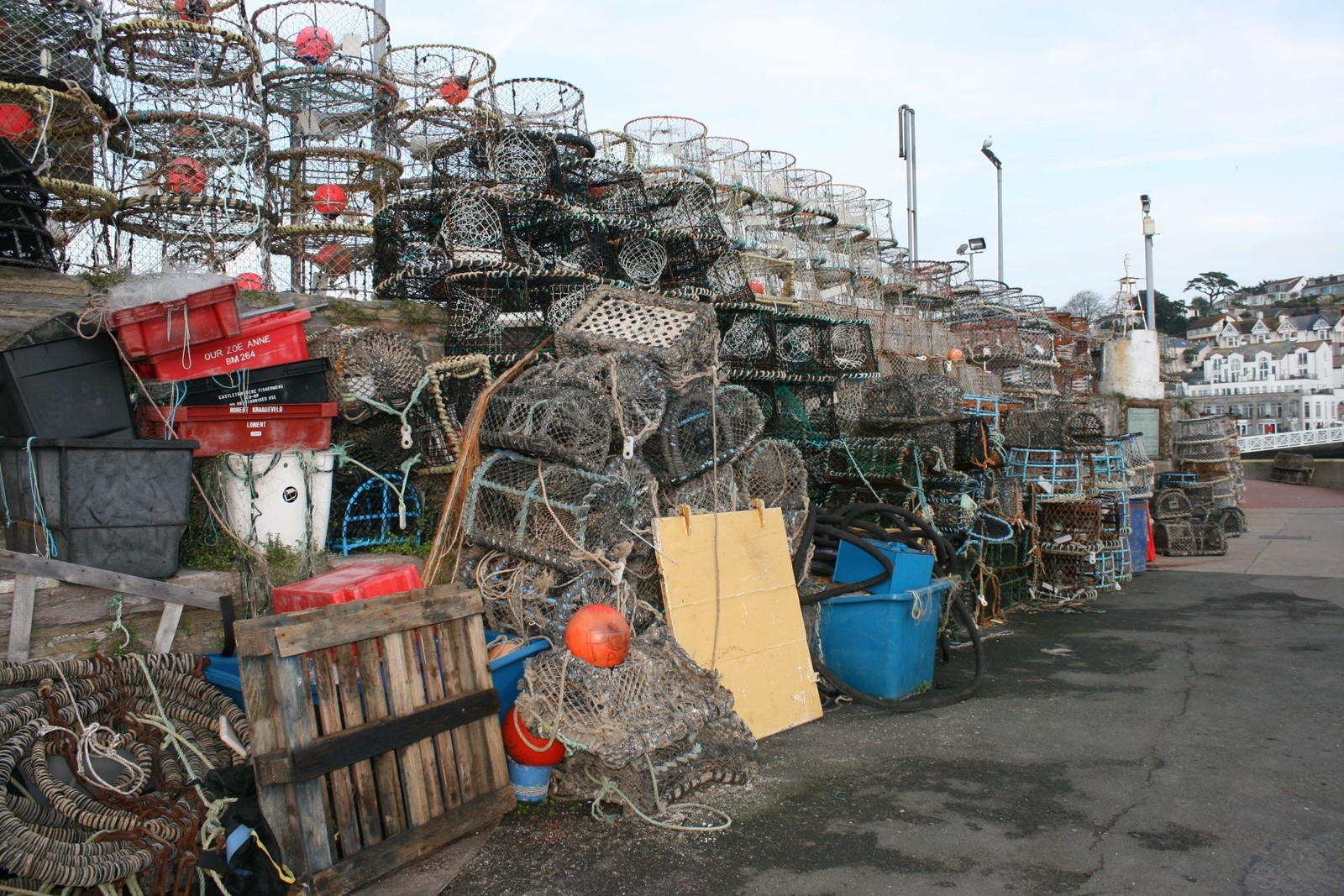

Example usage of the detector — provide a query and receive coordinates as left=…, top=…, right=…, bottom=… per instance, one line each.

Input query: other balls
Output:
left=565, top=604, right=630, bottom=667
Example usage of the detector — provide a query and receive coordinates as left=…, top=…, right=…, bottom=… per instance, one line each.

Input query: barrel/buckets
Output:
left=1116, top=499, right=1150, bottom=574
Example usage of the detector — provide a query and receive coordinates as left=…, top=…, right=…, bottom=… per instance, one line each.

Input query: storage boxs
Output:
left=273, top=564, right=441, bottom=670
left=1, top=330, right=137, bottom=439
left=107, top=281, right=242, bottom=361
left=1, top=436, right=200, bottom=579
left=195, top=626, right=553, bottom=730
left=804, top=575, right=956, bottom=700
left=214, top=454, right=335, bottom=555
left=132, top=307, right=312, bottom=384
left=166, top=356, right=333, bottom=406
left=1126, top=498, right=1148, bottom=575
left=833, top=538, right=936, bottom=595
left=132, top=403, right=338, bottom=454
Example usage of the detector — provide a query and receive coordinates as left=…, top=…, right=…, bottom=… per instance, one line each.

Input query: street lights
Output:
left=981, top=135, right=1003, bottom=284
